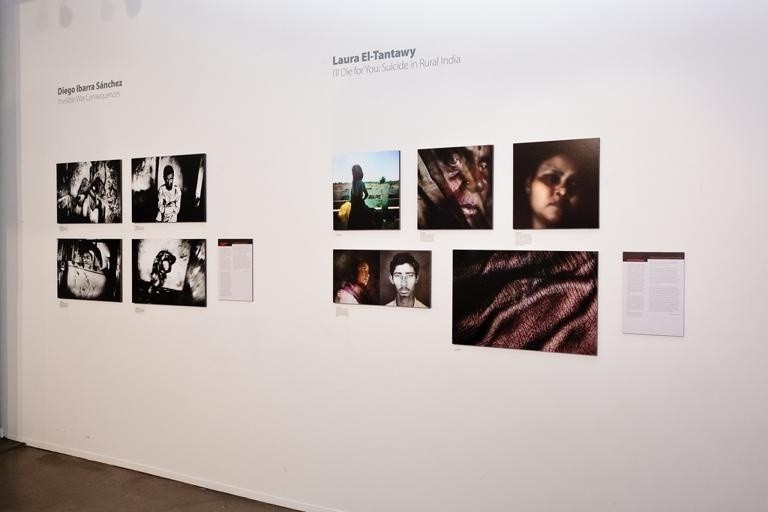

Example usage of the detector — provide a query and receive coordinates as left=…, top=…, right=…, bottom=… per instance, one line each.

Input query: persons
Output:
left=384, top=251, right=428, bottom=308
left=334, top=252, right=373, bottom=304
left=426, top=143, right=491, bottom=230
left=347, top=163, right=385, bottom=228
left=148, top=247, right=177, bottom=296
left=520, top=147, right=598, bottom=228
left=154, top=164, right=183, bottom=221
left=78, top=250, right=98, bottom=272
